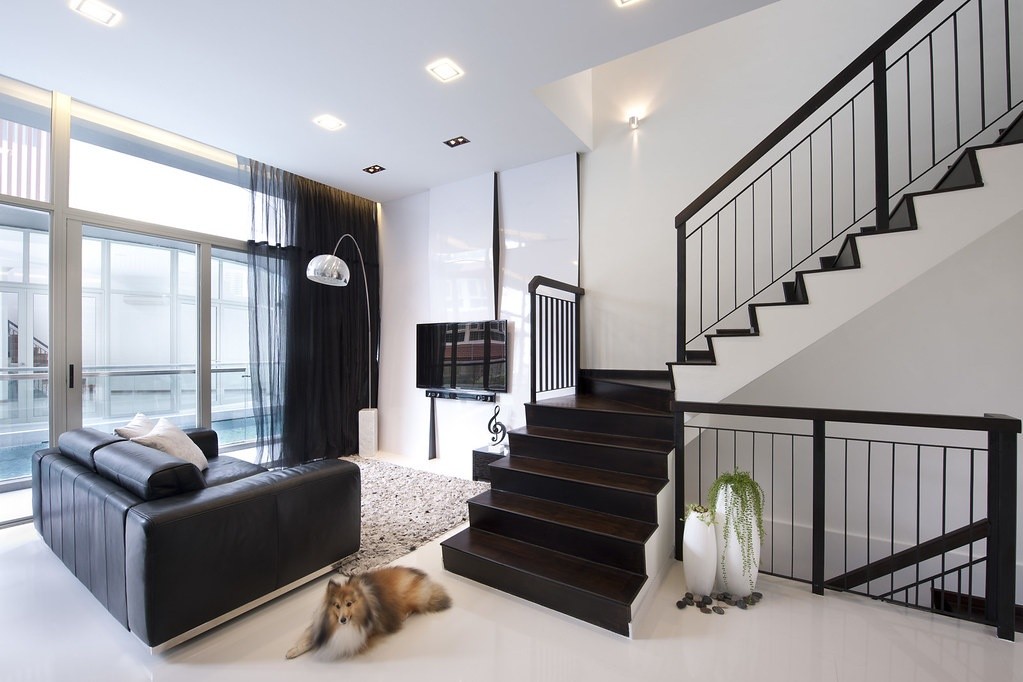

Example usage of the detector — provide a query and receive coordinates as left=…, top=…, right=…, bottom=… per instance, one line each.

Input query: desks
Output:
left=472, top=443, right=510, bottom=482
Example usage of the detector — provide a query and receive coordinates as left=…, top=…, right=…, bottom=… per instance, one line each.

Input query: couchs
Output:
left=31, top=427, right=361, bottom=656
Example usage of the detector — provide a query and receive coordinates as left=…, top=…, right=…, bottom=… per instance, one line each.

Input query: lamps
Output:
left=306, top=234, right=379, bottom=457
left=629, top=117, right=639, bottom=129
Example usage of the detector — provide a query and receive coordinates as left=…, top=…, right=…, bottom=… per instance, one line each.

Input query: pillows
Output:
left=114, top=412, right=153, bottom=438
left=129, top=417, right=209, bottom=472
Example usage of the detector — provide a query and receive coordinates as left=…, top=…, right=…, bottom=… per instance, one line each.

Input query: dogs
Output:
left=285, top=565, right=453, bottom=663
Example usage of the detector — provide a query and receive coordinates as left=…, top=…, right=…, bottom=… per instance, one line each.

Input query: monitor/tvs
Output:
left=416, top=320, right=508, bottom=394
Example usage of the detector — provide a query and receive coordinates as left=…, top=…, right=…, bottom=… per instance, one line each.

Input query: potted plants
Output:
left=679, top=504, right=717, bottom=597
left=710, top=466, right=767, bottom=599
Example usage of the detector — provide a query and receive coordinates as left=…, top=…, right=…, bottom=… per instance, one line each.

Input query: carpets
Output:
left=333, top=454, right=488, bottom=578
